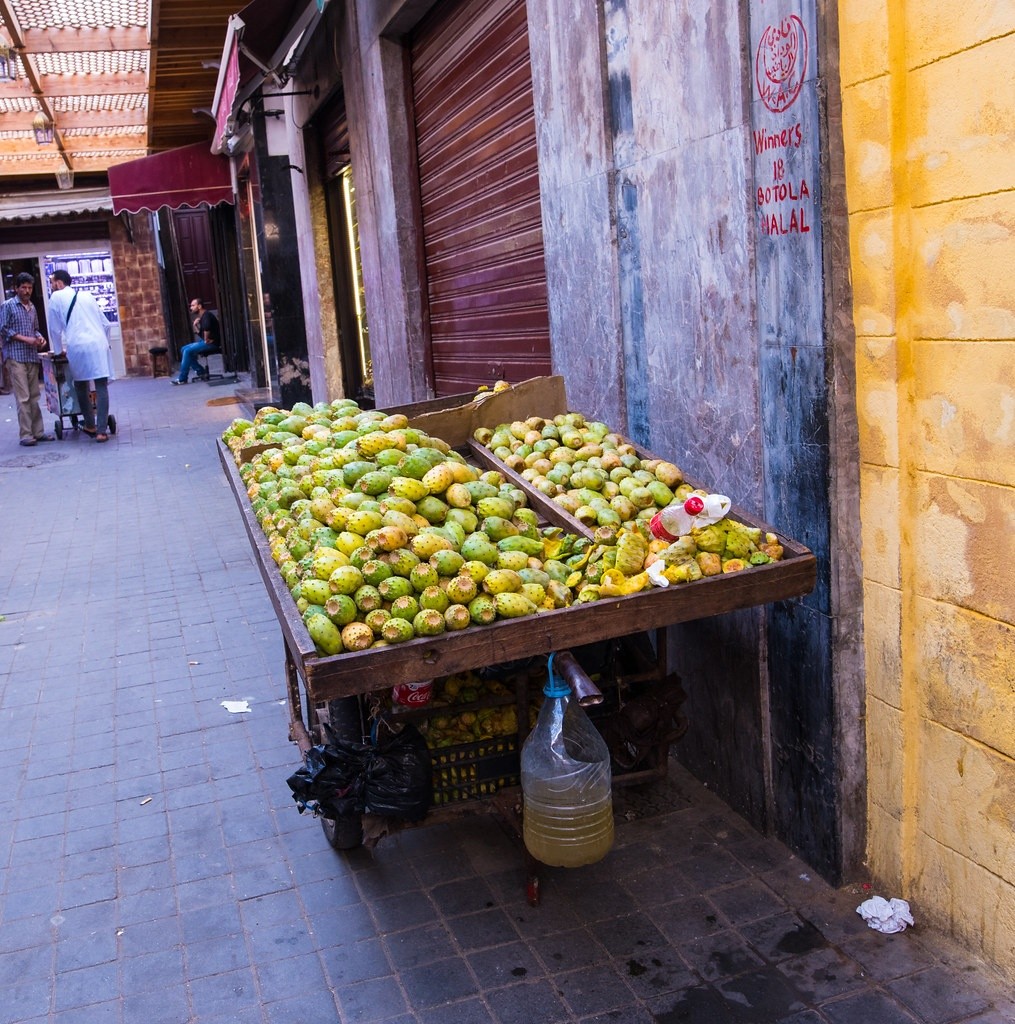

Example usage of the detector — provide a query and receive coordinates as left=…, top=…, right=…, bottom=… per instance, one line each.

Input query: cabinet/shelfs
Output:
left=44, top=250, right=127, bottom=379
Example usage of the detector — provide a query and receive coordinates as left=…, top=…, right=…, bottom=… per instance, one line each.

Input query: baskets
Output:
left=409, top=731, right=531, bottom=810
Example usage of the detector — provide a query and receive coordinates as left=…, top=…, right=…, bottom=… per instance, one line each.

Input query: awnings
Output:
left=108, top=137, right=236, bottom=217
left=189, top=1, right=325, bottom=151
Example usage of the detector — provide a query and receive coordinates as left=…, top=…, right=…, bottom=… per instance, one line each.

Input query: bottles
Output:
left=520, top=676, right=615, bottom=868
left=648, top=497, right=704, bottom=544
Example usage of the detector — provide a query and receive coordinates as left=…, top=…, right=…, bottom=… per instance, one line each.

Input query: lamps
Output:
left=33, top=98, right=54, bottom=146
left=56, top=152, right=74, bottom=190
left=0, top=34, right=18, bottom=83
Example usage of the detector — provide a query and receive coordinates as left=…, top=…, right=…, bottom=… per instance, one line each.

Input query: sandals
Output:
left=19, top=438, right=37, bottom=447
left=36, top=433, right=55, bottom=442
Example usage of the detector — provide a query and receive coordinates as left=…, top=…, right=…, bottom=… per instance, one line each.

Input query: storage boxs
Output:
left=369, top=665, right=622, bottom=810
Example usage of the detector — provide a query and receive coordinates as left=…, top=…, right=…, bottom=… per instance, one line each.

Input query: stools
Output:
left=150, top=346, right=170, bottom=379
left=192, top=350, right=224, bottom=383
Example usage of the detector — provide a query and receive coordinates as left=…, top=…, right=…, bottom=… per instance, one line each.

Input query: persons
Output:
left=170, top=298, right=222, bottom=385
left=46, top=269, right=112, bottom=442
left=255, top=292, right=274, bottom=355
left=0, top=272, right=56, bottom=447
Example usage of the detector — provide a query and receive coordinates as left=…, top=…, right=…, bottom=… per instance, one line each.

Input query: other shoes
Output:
left=170, top=379, right=187, bottom=386
left=192, top=373, right=209, bottom=382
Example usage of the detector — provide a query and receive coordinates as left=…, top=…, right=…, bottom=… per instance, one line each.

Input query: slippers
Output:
left=95, top=433, right=110, bottom=443
left=77, top=420, right=98, bottom=438
